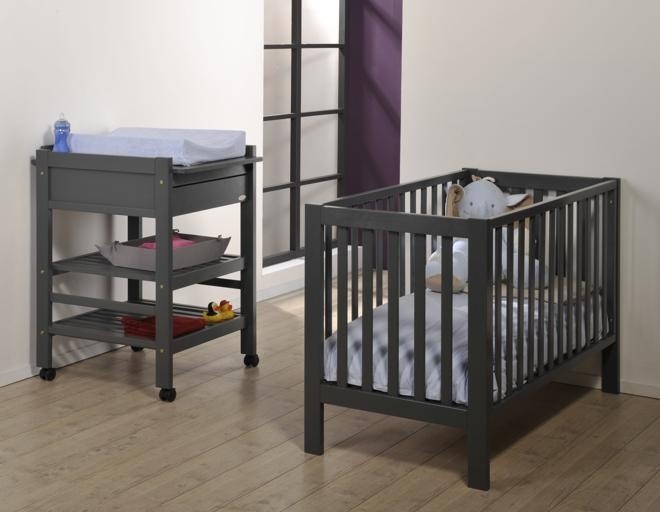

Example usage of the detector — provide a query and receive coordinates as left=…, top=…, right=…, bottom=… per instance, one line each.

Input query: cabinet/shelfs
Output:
left=32, top=144, right=256, bottom=388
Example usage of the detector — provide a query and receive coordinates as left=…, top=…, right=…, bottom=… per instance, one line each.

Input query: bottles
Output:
left=52, top=112, right=70, bottom=154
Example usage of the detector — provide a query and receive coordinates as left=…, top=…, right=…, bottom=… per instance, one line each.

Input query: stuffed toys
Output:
left=423, top=177, right=550, bottom=294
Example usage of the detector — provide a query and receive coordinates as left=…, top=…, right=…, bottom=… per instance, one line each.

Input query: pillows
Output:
left=463, top=274, right=599, bottom=305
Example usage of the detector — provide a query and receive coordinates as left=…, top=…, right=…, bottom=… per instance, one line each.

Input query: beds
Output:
left=306, top=168, right=619, bottom=490
